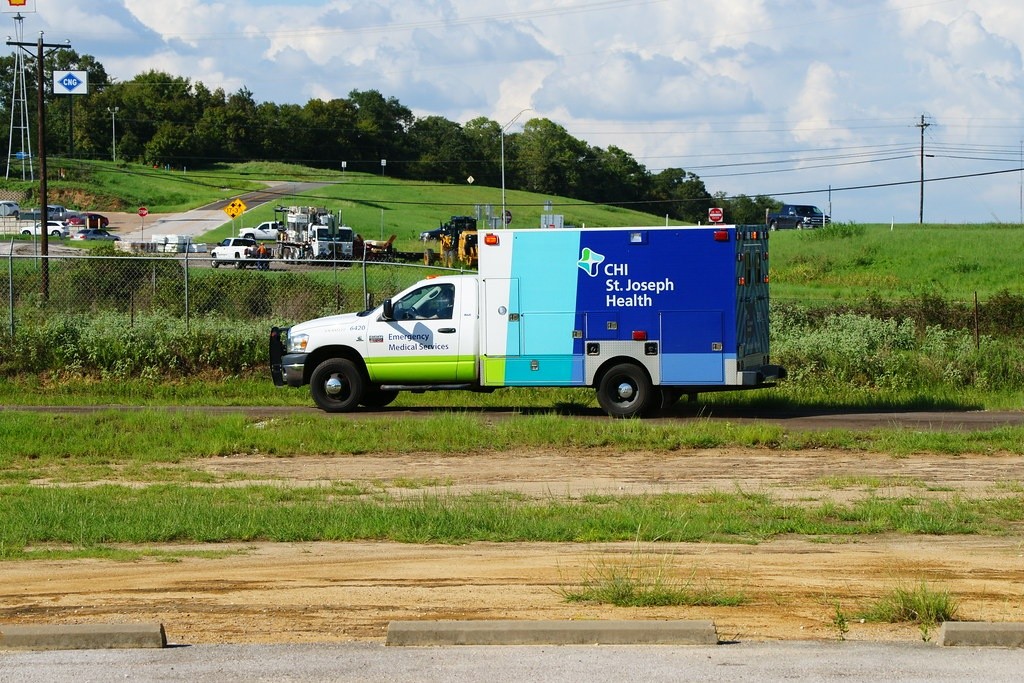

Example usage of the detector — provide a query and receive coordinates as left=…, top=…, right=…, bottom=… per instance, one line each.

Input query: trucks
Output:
left=269, top=223, right=772, bottom=419
left=275, top=207, right=356, bottom=266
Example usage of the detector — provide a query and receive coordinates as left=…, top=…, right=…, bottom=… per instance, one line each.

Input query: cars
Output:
left=65, top=213, right=109, bottom=227
left=238, top=222, right=284, bottom=240
left=48, top=204, right=80, bottom=221
left=70, top=227, right=121, bottom=241
left=18, top=220, right=70, bottom=238
left=0, top=201, right=19, bottom=216
left=769, top=204, right=831, bottom=231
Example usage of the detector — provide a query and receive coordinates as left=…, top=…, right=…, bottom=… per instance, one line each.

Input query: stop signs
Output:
left=138, top=207, right=147, bottom=217
left=709, top=208, right=722, bottom=223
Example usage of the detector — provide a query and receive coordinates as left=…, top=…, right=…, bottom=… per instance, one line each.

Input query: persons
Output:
left=407, top=289, right=453, bottom=319
left=256, top=241, right=269, bottom=270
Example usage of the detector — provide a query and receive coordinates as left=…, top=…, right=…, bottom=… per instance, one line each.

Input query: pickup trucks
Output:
left=211, top=236, right=272, bottom=269
